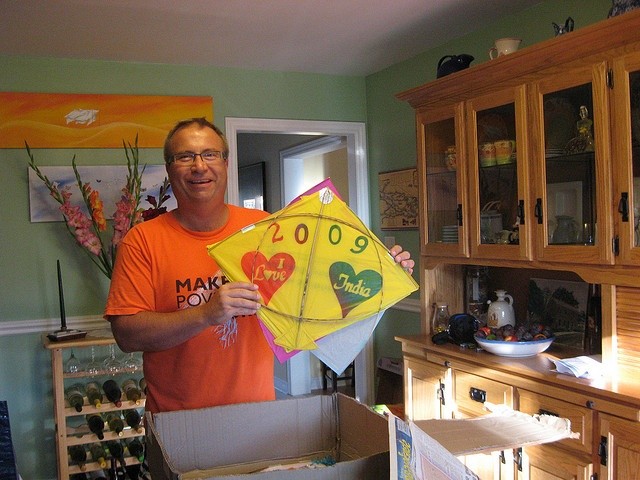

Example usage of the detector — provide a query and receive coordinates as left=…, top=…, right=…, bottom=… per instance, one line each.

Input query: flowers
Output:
left=24, top=130, right=170, bottom=280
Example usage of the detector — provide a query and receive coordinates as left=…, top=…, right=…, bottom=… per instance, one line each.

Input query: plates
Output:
left=473, top=334, right=556, bottom=357
left=442, top=225, right=458, bottom=242
left=545, top=149, right=561, bottom=157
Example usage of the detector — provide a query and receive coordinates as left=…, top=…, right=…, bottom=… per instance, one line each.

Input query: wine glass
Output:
left=125, top=352, right=142, bottom=376
left=88, top=345, right=103, bottom=377
left=65, top=347, right=84, bottom=372
left=105, top=344, right=124, bottom=377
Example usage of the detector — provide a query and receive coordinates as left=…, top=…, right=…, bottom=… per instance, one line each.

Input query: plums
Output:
left=476, top=323, right=552, bottom=342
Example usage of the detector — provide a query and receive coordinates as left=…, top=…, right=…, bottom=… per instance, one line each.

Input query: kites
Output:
left=204, top=180, right=421, bottom=365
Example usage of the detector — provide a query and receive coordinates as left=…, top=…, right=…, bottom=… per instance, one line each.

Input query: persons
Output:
left=102, top=115, right=416, bottom=479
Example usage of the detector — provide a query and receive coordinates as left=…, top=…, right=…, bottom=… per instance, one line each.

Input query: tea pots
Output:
left=508, top=229, right=519, bottom=244
left=487, top=289, right=516, bottom=329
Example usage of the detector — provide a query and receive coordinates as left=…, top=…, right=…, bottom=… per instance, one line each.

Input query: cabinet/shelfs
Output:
left=451, top=366, right=512, bottom=480
left=598, top=413, right=640, bottom=480
left=403, top=356, right=452, bottom=422
left=465, top=59, right=609, bottom=268
left=609, top=49, right=640, bottom=266
left=418, top=101, right=469, bottom=260
left=512, top=387, right=597, bottom=480
left=40, top=328, right=148, bottom=479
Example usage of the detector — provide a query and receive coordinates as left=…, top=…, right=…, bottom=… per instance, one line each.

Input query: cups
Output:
left=489, top=37, right=520, bottom=60
left=495, top=140, right=516, bottom=165
left=478, top=141, right=496, bottom=167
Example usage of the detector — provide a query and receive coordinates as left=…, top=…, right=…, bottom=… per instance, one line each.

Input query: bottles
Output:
left=102, top=380, right=122, bottom=408
left=120, top=461, right=127, bottom=480
left=139, top=377, right=149, bottom=395
left=67, top=445, right=87, bottom=471
left=86, top=412, right=104, bottom=440
left=90, top=470, right=106, bottom=480
left=124, top=437, right=144, bottom=462
left=105, top=410, right=124, bottom=437
left=586, top=224, right=594, bottom=245
left=433, top=305, right=449, bottom=335
left=122, top=378, right=141, bottom=406
left=138, top=463, right=152, bottom=480
left=86, top=382, right=102, bottom=409
left=107, top=439, right=126, bottom=465
left=88, top=442, right=107, bottom=468
left=122, top=409, right=142, bottom=433
left=109, top=457, right=119, bottom=480
left=66, top=386, right=84, bottom=412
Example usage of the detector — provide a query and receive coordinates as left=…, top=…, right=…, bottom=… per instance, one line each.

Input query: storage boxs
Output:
left=148, top=387, right=582, bottom=479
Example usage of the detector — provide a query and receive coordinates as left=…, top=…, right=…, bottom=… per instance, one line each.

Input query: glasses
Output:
left=167, top=151, right=223, bottom=165
